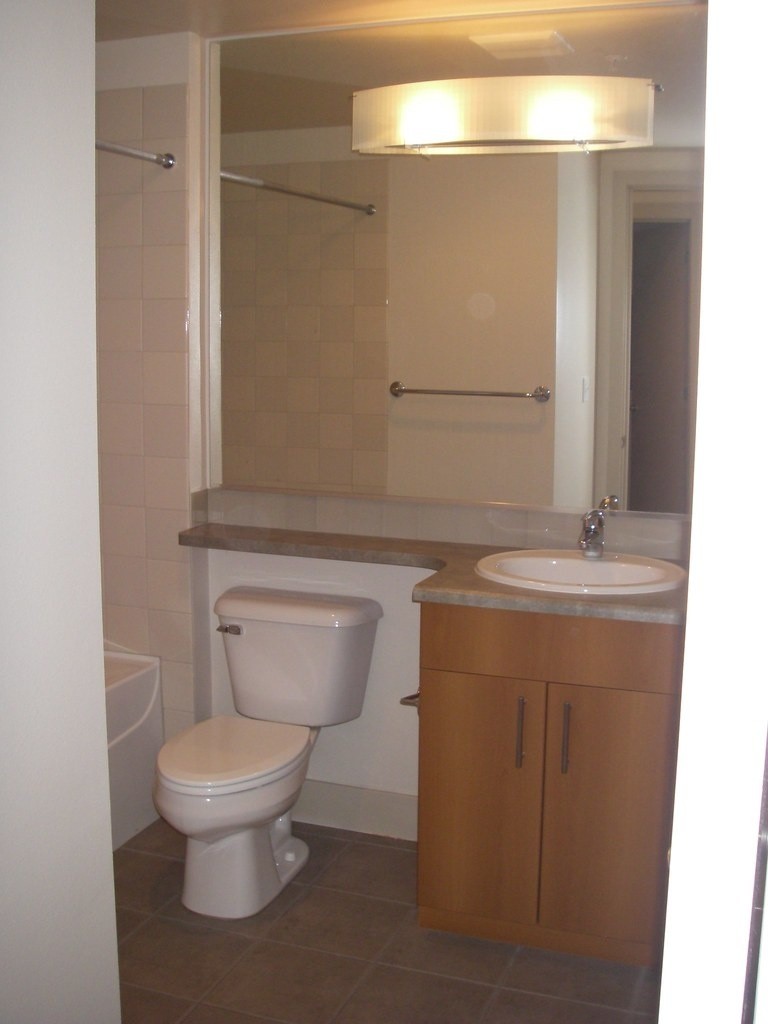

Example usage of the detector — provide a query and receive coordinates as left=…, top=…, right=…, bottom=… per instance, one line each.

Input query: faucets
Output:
left=597, top=494, right=621, bottom=513
left=575, top=509, right=607, bottom=560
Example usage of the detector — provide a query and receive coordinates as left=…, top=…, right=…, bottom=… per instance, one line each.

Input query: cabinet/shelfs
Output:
left=411, top=555, right=684, bottom=969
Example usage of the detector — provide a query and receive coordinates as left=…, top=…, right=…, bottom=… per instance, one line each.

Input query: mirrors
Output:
left=193, top=2, right=709, bottom=561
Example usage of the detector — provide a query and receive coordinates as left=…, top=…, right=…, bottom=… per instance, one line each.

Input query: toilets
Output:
left=148, top=583, right=385, bottom=921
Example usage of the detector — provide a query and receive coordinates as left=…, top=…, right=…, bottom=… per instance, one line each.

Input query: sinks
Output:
left=471, top=544, right=689, bottom=598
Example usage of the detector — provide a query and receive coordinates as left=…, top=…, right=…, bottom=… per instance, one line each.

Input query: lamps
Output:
left=350, top=75, right=657, bottom=155
